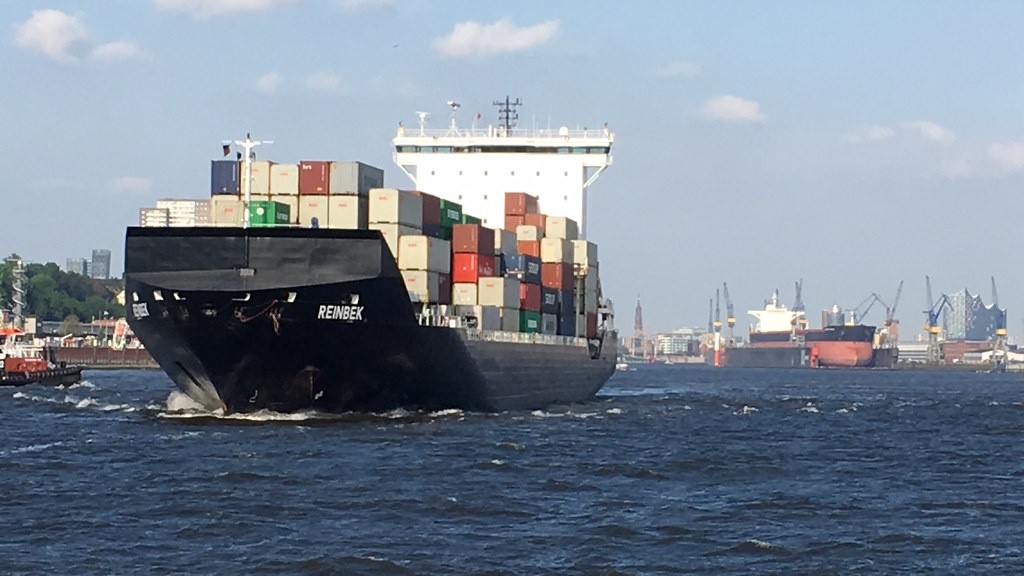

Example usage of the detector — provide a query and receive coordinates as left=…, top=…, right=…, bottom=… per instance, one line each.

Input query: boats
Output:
left=121, top=88, right=622, bottom=408
left=0, top=259, right=85, bottom=387
